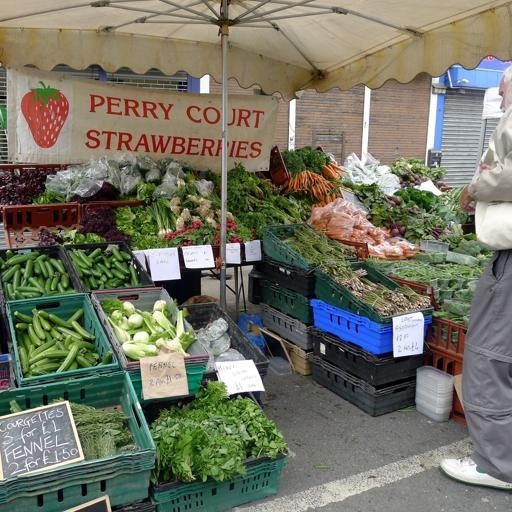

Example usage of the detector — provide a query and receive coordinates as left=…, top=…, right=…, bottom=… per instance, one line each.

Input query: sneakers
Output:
left=440, top=459, right=512, bottom=491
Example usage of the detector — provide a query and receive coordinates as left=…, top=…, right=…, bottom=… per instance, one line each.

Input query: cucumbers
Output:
left=13, top=308, right=113, bottom=378
left=0, top=247, right=139, bottom=301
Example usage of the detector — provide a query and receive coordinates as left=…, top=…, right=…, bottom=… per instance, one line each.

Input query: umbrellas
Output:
left=1, top=0, right=511, bottom=312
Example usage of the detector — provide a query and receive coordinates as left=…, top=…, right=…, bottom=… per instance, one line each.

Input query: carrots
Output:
left=285, top=163, right=420, bottom=261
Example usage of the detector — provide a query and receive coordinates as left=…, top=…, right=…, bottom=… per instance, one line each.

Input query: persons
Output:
left=438, top=64, right=511, bottom=492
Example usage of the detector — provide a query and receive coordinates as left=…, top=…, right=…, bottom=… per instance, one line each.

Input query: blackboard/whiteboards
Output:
left=63, top=494, right=112, bottom=512
left=0, top=400, right=85, bottom=480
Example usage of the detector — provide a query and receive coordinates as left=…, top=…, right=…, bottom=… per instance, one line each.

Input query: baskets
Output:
left=0, top=202, right=287, bottom=512
left=309, top=260, right=465, bottom=417
left=259, top=222, right=467, bottom=428
left=259, top=223, right=369, bottom=376
left=1, top=202, right=287, bottom=512
